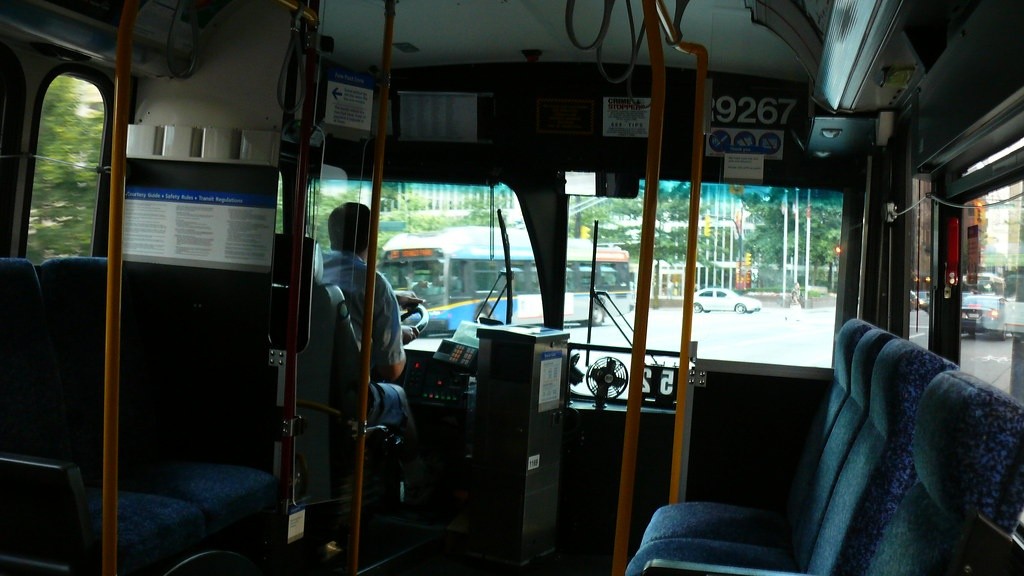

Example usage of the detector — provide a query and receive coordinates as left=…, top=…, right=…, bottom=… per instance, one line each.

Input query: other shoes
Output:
left=399, top=454, right=433, bottom=504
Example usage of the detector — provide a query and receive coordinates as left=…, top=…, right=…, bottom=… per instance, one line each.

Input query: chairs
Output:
left=295, top=240, right=400, bottom=500
left=37, top=256, right=280, bottom=536
left=0, top=258, right=208, bottom=576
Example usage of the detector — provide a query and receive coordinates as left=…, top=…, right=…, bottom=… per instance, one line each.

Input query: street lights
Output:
left=723, top=216, right=743, bottom=296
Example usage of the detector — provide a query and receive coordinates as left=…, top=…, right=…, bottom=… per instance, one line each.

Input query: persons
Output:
left=785, top=282, right=803, bottom=321
left=321, top=202, right=440, bottom=505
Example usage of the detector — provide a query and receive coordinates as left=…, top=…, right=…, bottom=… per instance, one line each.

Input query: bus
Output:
left=961, top=273, right=1006, bottom=298
left=378, top=225, right=636, bottom=330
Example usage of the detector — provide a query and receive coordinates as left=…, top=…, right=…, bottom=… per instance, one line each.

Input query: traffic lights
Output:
left=974, top=201, right=986, bottom=226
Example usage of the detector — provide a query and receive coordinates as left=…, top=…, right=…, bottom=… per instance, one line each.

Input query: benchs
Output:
left=620, top=319, right=1022, bottom=576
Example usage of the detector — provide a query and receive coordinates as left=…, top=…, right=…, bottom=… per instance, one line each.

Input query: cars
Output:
left=909, top=290, right=932, bottom=312
left=693, top=288, right=762, bottom=314
left=960, top=295, right=1016, bottom=341
